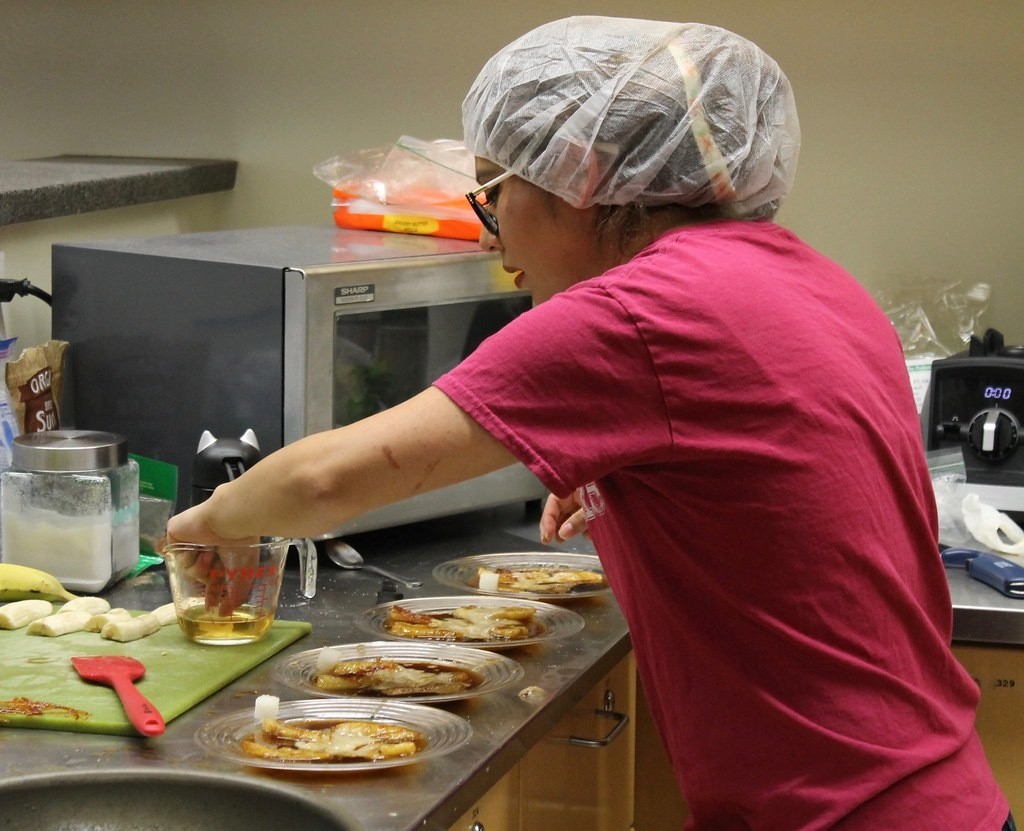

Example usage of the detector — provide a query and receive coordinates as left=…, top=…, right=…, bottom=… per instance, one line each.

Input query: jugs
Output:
left=152, top=534, right=319, bottom=646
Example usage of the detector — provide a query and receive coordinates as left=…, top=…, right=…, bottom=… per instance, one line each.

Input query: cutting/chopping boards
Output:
left=0, top=604, right=311, bottom=736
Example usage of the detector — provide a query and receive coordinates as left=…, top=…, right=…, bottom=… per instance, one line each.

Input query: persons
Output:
left=162, top=12, right=1017, bottom=831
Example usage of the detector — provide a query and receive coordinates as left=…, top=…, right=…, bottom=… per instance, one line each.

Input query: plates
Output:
left=273, top=640, right=524, bottom=704
left=433, top=552, right=614, bottom=604
left=194, top=699, right=474, bottom=775
left=355, top=595, right=585, bottom=651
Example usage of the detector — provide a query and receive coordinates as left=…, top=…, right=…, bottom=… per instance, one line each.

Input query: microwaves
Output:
left=52, top=225, right=553, bottom=542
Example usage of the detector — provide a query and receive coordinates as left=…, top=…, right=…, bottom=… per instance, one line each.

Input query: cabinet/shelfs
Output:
left=444, top=642, right=637, bottom=831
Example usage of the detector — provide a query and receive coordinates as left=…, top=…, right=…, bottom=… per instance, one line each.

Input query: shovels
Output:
left=70, top=654, right=165, bottom=737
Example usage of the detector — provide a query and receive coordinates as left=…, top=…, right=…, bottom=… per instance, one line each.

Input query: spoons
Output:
left=326, top=542, right=422, bottom=588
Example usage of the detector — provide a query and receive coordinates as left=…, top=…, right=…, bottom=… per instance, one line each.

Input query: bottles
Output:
left=0, top=430, right=139, bottom=595
